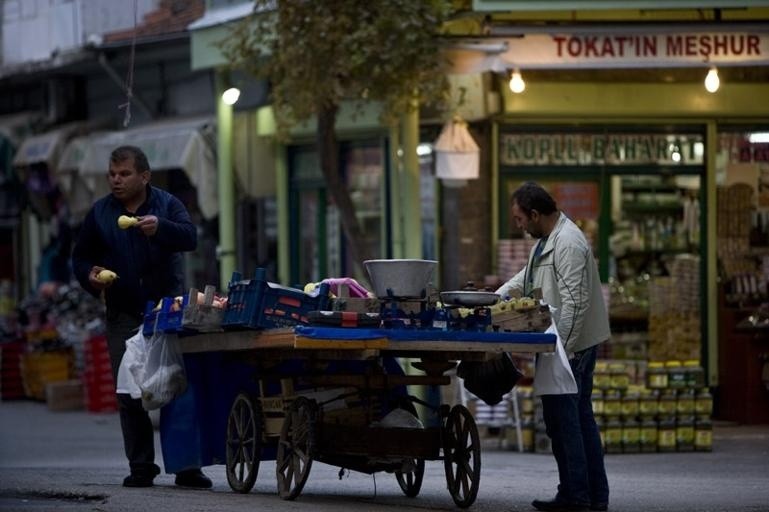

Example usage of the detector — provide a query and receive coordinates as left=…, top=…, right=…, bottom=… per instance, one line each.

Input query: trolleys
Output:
left=161, top=329, right=559, bottom=509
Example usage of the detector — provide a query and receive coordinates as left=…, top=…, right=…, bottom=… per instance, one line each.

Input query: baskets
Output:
left=220, top=279, right=330, bottom=329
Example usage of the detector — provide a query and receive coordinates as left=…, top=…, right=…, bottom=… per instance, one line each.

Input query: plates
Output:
left=439, top=290, right=500, bottom=306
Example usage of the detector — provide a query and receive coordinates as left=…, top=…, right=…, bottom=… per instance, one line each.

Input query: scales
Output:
left=363, top=259, right=502, bottom=333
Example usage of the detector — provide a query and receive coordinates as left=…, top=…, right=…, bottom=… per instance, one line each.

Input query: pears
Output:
left=97, top=269, right=119, bottom=283
left=117, top=215, right=140, bottom=230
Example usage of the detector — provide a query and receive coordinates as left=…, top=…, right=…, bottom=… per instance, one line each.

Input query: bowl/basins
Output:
left=362, top=258, right=441, bottom=298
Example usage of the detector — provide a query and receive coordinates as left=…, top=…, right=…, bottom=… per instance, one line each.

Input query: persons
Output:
left=493, top=182, right=613, bottom=511
left=71, top=144, right=215, bottom=492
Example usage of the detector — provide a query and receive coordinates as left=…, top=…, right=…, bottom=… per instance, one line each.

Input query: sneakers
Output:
left=123, top=474, right=155, bottom=487
left=176, top=473, right=213, bottom=489
left=532, top=497, right=608, bottom=511
